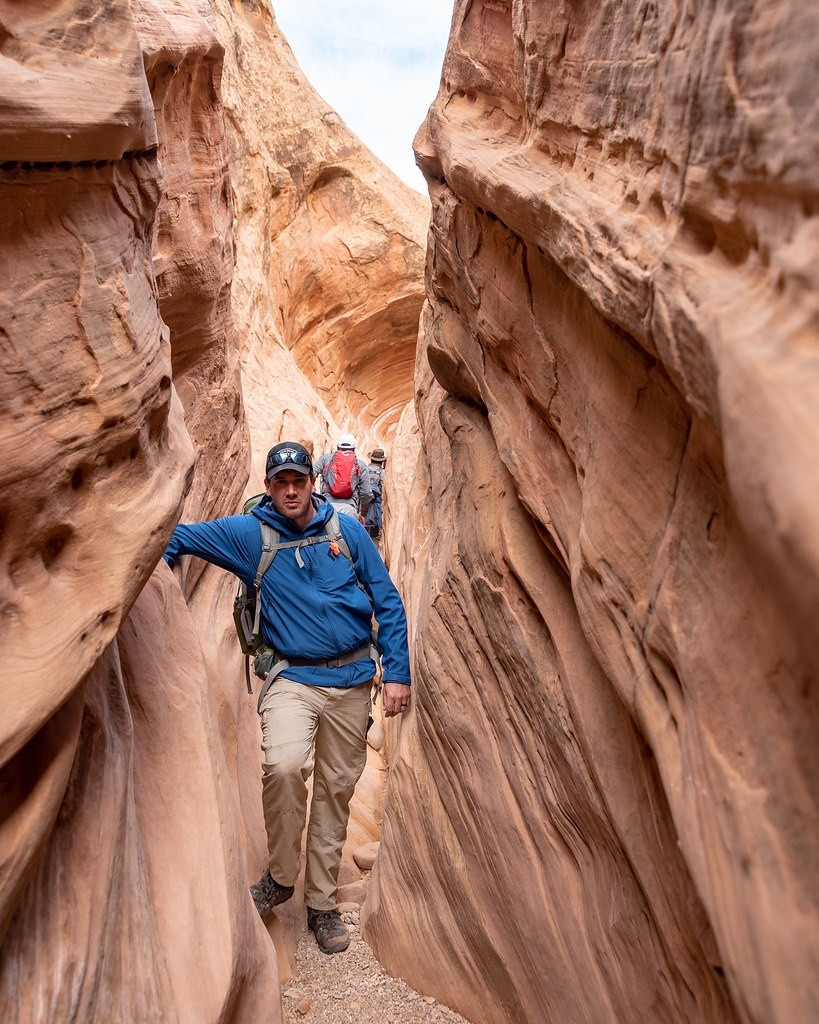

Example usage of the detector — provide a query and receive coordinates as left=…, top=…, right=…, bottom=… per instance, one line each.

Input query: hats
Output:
left=338, top=443, right=355, bottom=449
left=367, top=449, right=386, bottom=461
left=266, top=442, right=313, bottom=480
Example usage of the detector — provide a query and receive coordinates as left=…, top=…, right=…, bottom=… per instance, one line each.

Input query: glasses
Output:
left=266, top=452, right=311, bottom=473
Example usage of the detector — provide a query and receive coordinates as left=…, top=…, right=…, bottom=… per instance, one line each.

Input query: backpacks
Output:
left=370, top=467, right=382, bottom=499
left=323, top=450, right=361, bottom=498
left=233, top=492, right=354, bottom=680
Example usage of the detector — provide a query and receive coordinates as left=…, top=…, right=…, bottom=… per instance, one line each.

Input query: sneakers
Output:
left=308, top=906, right=349, bottom=954
left=250, top=869, right=294, bottom=916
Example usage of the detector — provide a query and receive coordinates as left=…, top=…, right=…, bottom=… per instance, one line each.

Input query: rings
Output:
left=401, top=703, right=407, bottom=706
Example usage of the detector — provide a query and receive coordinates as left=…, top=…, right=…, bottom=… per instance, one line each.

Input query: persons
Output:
left=312, top=434, right=374, bottom=527
left=162, top=440, right=412, bottom=953
left=364, top=448, right=387, bottom=538
